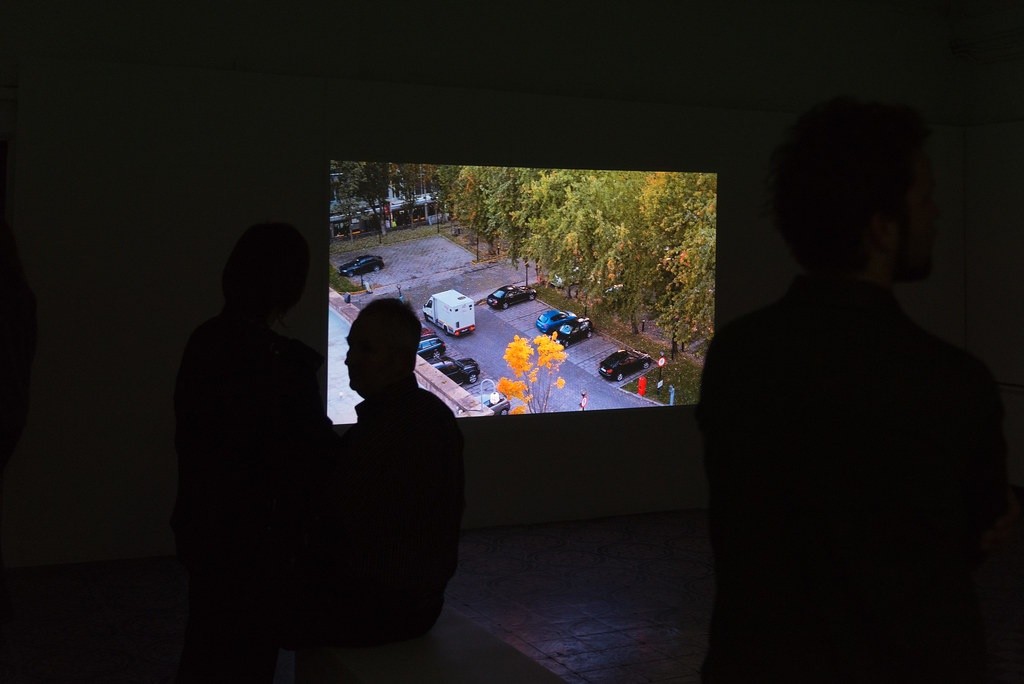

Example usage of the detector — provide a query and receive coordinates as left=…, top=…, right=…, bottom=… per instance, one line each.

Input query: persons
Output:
left=177, top=222, right=337, bottom=684
left=296, top=297, right=464, bottom=642
left=690, top=101, right=1018, bottom=684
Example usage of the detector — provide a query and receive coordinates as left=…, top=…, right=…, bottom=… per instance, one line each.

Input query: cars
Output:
left=472, top=393, right=509, bottom=417
left=338, top=255, right=384, bottom=278
left=548, top=265, right=581, bottom=289
left=418, top=328, right=433, bottom=340
left=487, top=284, right=538, bottom=311
left=598, top=348, right=653, bottom=383
left=549, top=316, right=592, bottom=350
left=536, top=309, right=577, bottom=335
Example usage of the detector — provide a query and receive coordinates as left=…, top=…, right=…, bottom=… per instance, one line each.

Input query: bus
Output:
left=330, top=190, right=451, bottom=244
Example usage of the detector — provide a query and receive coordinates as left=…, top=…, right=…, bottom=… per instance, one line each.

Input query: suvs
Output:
left=415, top=334, right=445, bottom=360
left=426, top=356, right=480, bottom=389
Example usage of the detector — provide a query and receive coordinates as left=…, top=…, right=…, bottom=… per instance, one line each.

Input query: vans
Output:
left=422, top=290, right=476, bottom=336
